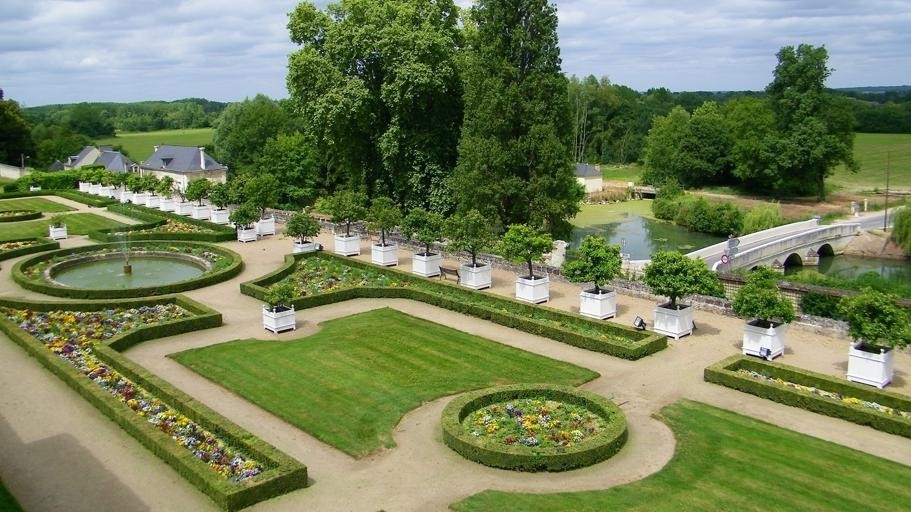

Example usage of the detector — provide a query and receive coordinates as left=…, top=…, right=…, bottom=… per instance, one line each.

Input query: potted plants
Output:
left=399, top=205, right=443, bottom=278
left=183, top=177, right=210, bottom=221
left=282, top=211, right=321, bottom=254
left=492, top=221, right=553, bottom=305
left=260, top=282, right=295, bottom=335
left=173, top=180, right=193, bottom=215
left=142, top=171, right=159, bottom=208
left=835, top=285, right=909, bottom=391
left=228, top=183, right=261, bottom=243
left=441, top=207, right=492, bottom=290
left=252, top=184, right=275, bottom=237
left=28, top=170, right=41, bottom=191
left=76, top=167, right=132, bottom=203
left=126, top=172, right=145, bottom=205
left=364, top=196, right=401, bottom=268
left=48, top=212, right=67, bottom=242
left=314, top=190, right=365, bottom=257
left=641, top=249, right=726, bottom=342
left=209, top=180, right=236, bottom=225
left=732, top=264, right=797, bottom=364
left=562, top=233, right=621, bottom=322
left=156, top=175, right=175, bottom=212
